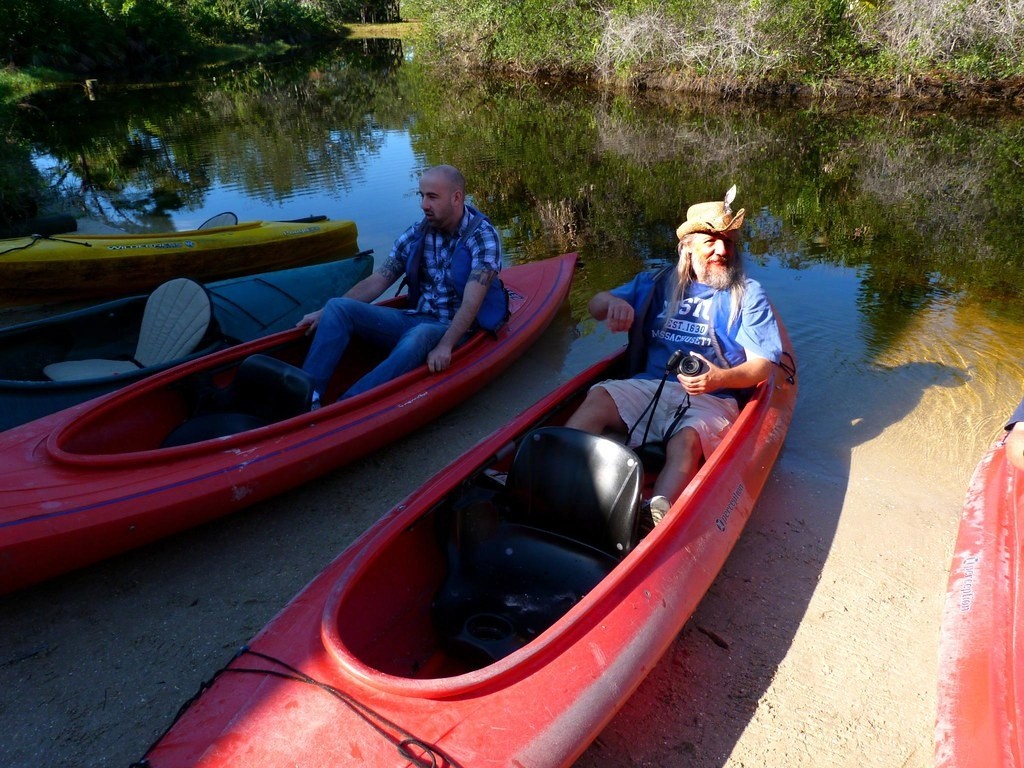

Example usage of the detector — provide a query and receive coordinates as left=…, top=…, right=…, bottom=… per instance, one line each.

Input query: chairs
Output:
left=160, top=355, right=316, bottom=450
left=43, top=277, right=214, bottom=381
left=195, top=211, right=237, bottom=230
left=428, top=427, right=644, bottom=672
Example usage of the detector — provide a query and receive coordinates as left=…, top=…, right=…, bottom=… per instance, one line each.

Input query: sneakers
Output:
left=311, top=390, right=320, bottom=412
left=485, top=467, right=507, bottom=486
left=641, top=494, right=672, bottom=528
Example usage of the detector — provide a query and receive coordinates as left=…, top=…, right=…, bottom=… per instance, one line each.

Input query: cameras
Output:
left=667, top=349, right=710, bottom=377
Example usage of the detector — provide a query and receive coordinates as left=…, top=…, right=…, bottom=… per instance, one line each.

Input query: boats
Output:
left=935, top=422, right=1024, bottom=768
left=128, top=286, right=797, bottom=768
left=0, top=219, right=357, bottom=318
left=1, top=252, right=577, bottom=599
left=0, top=251, right=376, bottom=434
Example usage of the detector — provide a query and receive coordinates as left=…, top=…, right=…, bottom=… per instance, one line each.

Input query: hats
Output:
left=675, top=201, right=746, bottom=239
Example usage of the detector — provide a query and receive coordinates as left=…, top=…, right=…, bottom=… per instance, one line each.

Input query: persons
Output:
left=296, top=164, right=510, bottom=409
left=564, top=183, right=782, bottom=538
